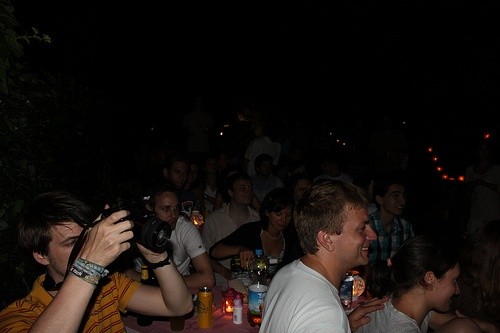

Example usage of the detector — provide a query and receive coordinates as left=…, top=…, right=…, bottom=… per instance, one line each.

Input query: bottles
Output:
left=233, top=293, right=244, bottom=325
left=247, top=249, right=268, bottom=328
left=230, top=256, right=282, bottom=271
left=339, top=270, right=352, bottom=309
left=137, top=264, right=153, bottom=325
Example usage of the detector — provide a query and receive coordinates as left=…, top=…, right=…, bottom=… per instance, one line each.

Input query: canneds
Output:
left=197, top=286, right=212, bottom=328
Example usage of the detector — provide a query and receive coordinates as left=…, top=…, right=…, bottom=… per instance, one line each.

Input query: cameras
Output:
left=101, top=196, right=171, bottom=251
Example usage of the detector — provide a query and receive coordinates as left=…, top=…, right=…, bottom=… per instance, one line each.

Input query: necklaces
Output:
left=265, top=231, right=282, bottom=240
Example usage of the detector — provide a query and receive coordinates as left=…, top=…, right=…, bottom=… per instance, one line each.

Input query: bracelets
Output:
left=73, top=261, right=101, bottom=280
left=77, top=258, right=108, bottom=276
left=149, top=255, right=170, bottom=269
left=70, top=266, right=98, bottom=286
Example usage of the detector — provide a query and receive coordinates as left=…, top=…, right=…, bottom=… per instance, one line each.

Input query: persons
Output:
left=0, top=123, right=500, bottom=333
